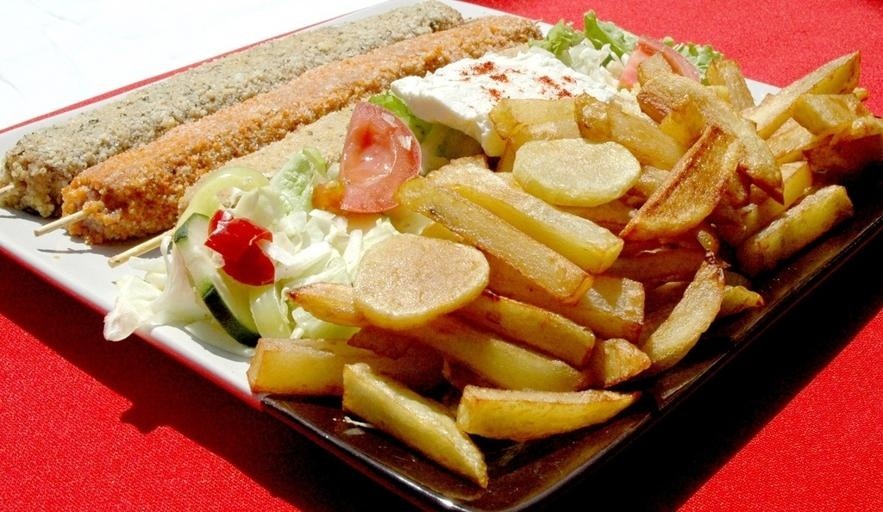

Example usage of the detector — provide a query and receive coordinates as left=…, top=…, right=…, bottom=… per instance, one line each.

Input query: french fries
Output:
left=245, top=8, right=882, bottom=490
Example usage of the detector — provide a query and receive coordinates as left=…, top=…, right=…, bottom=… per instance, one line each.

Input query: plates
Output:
left=0, top=0, right=883, bottom=511
